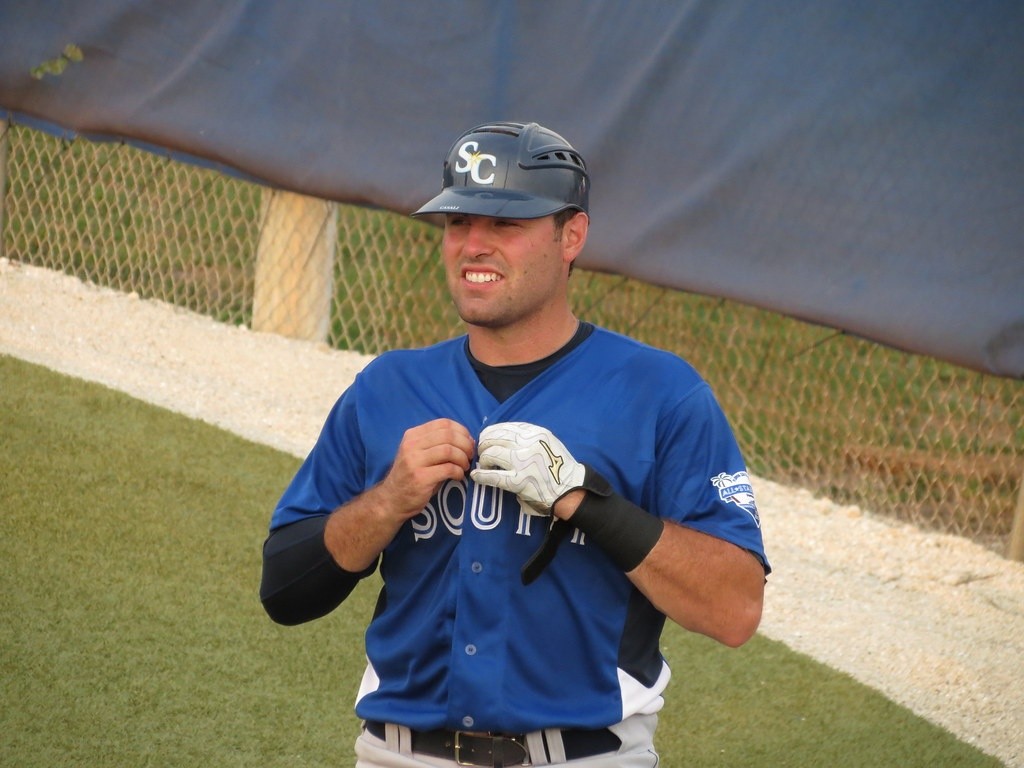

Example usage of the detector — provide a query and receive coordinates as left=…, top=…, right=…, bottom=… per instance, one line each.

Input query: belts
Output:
left=366, top=718, right=622, bottom=767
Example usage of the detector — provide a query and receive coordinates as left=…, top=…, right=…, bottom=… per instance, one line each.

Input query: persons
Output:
left=260, top=121, right=771, bottom=767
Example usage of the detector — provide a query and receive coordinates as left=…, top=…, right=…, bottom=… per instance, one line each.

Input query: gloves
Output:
left=471, top=421, right=614, bottom=586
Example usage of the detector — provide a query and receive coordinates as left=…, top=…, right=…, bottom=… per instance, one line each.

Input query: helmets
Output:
left=410, top=122, right=590, bottom=224
